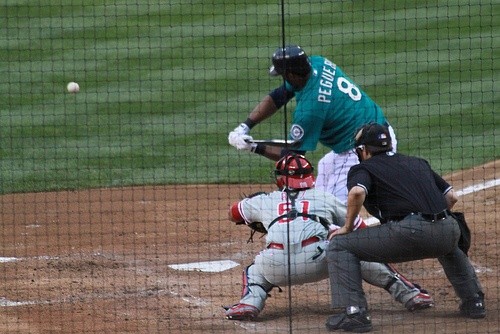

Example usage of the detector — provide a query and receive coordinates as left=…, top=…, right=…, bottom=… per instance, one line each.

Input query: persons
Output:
left=228, top=46, right=398, bottom=207
left=225, top=155, right=433, bottom=320
left=324, top=124, right=486, bottom=332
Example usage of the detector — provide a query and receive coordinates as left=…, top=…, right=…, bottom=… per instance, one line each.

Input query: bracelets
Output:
left=242, top=118, right=255, bottom=129
left=255, top=143, right=266, bottom=155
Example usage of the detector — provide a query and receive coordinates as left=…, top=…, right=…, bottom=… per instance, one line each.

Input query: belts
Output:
left=410, top=210, right=451, bottom=222
left=268, top=237, right=321, bottom=249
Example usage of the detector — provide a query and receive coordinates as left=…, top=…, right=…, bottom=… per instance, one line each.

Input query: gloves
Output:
left=227, top=124, right=257, bottom=156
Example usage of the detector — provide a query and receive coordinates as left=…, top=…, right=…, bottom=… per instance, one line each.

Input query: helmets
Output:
left=274, top=156, right=316, bottom=188
left=270, top=45, right=309, bottom=77
left=353, top=123, right=393, bottom=154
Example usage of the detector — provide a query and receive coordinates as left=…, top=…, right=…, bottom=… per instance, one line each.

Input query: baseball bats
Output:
left=246, top=138, right=302, bottom=149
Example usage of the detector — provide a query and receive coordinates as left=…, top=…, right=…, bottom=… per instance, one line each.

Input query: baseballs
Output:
left=67, top=82, right=79, bottom=94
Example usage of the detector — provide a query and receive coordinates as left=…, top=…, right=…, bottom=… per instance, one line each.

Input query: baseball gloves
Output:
left=237, top=192, right=268, bottom=233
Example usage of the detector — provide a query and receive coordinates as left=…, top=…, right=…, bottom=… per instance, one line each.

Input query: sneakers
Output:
left=325, top=311, right=372, bottom=333
left=407, top=291, right=434, bottom=310
left=224, top=305, right=260, bottom=321
left=460, top=297, right=487, bottom=317
